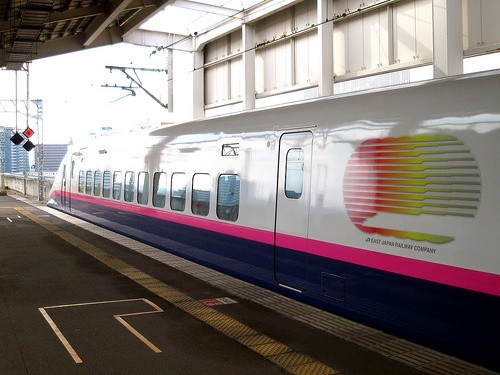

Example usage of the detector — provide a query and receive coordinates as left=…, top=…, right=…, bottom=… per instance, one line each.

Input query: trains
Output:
left=44, top=117, right=500, bottom=370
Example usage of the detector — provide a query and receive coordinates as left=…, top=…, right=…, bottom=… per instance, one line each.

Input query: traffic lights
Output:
left=9, top=127, right=35, bottom=151
